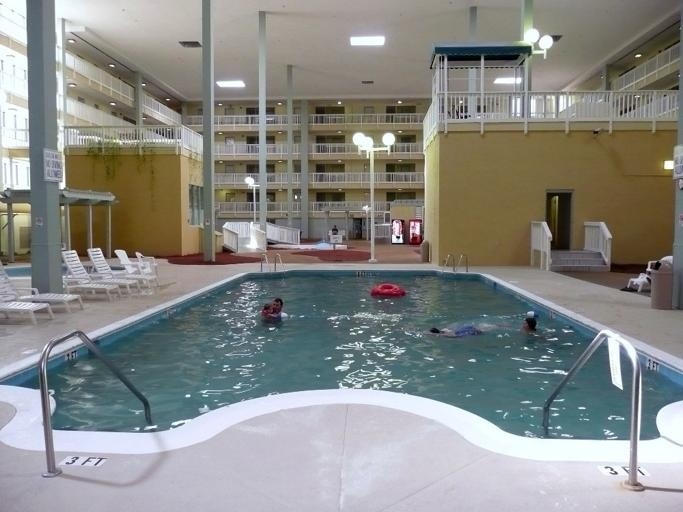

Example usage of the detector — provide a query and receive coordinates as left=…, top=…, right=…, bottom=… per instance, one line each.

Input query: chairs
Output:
left=0, top=249, right=167, bottom=324
left=627, top=254, right=676, bottom=292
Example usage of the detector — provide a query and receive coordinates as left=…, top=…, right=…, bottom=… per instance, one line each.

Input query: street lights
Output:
left=245, top=176, right=260, bottom=223
left=353, top=133, right=396, bottom=263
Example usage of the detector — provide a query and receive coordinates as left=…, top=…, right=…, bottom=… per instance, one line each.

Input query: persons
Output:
left=430, top=324, right=511, bottom=338
left=332, top=225, right=338, bottom=235
left=262, top=299, right=283, bottom=323
left=522, top=318, right=537, bottom=333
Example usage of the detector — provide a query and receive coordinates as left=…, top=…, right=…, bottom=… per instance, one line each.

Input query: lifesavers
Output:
left=370, top=283, right=407, bottom=297
